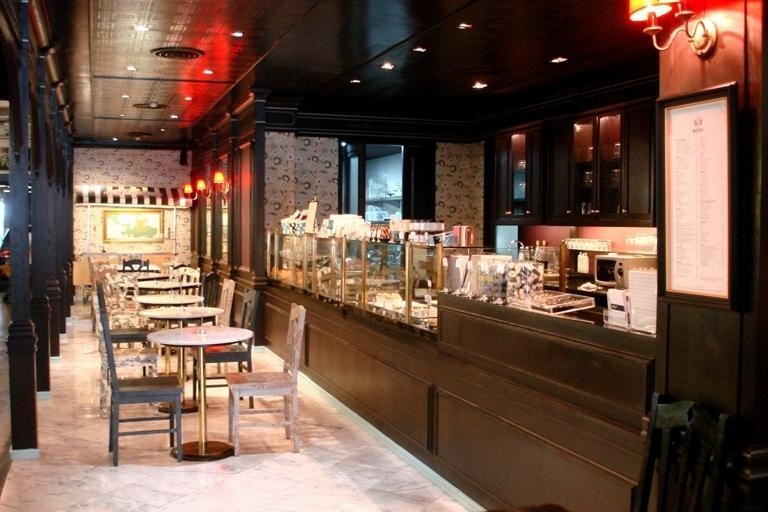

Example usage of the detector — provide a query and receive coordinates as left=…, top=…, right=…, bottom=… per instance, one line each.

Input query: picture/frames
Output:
left=102, top=209, right=164, bottom=243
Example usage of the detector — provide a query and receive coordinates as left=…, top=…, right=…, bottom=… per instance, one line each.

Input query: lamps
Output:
left=182, top=172, right=231, bottom=209
left=629, top=0, right=717, bottom=58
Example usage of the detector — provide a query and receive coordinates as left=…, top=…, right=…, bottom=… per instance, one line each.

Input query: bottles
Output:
left=577, top=251, right=589, bottom=273
left=533, top=240, right=548, bottom=263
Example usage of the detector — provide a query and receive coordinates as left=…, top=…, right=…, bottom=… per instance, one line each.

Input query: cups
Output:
left=453, top=225, right=474, bottom=247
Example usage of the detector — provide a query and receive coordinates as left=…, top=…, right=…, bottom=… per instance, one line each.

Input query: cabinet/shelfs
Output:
left=266, top=230, right=528, bottom=343
left=490, top=92, right=658, bottom=227
left=365, top=195, right=403, bottom=225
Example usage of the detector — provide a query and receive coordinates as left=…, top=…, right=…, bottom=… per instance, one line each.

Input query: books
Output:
left=627, top=271, right=658, bottom=335
left=606, top=288, right=628, bottom=327
left=509, top=289, right=595, bottom=315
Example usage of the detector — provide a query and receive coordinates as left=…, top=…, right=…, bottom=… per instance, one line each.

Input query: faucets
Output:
left=508, top=240, right=525, bottom=254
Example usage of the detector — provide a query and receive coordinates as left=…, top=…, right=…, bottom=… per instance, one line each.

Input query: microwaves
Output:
left=593, top=253, right=657, bottom=290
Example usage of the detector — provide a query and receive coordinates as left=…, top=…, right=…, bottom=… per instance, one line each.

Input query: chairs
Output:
left=632, top=390, right=768, bottom=512
left=84, top=251, right=307, bottom=466
